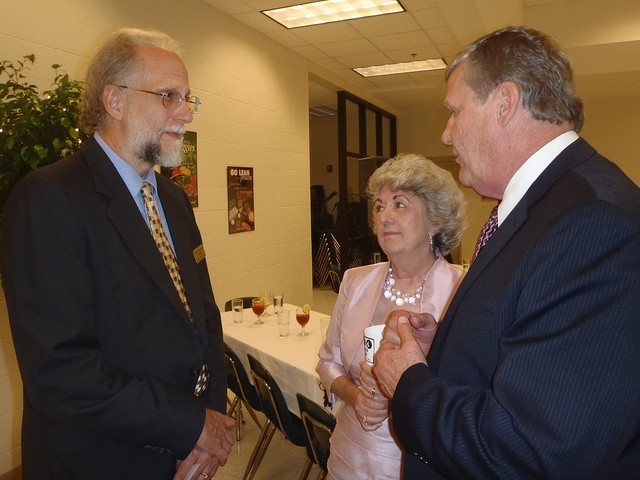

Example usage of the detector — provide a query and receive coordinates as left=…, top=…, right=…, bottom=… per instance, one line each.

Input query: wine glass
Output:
left=252, top=299, right=265, bottom=325
left=296, top=308, right=310, bottom=336
left=259, top=291, right=271, bottom=317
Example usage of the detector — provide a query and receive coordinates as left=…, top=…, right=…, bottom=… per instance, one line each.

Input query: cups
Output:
left=231, top=299, right=243, bottom=323
left=277, top=308, right=290, bottom=336
left=319, top=318, right=331, bottom=344
left=273, top=292, right=283, bottom=314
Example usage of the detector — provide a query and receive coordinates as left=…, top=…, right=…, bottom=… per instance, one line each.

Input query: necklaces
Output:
left=383, top=268, right=426, bottom=307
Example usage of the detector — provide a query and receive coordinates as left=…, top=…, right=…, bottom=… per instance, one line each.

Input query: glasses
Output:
left=114, top=84, right=201, bottom=114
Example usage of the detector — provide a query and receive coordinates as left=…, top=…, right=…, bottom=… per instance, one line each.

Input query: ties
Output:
left=139, top=181, right=210, bottom=398
left=469, top=203, right=498, bottom=269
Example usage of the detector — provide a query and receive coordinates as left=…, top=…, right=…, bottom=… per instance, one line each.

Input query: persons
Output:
left=0, top=29, right=236, bottom=480
left=315, top=154, right=470, bottom=480
left=372, top=25, right=638, bottom=480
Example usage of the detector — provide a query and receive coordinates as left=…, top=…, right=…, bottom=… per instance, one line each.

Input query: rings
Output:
left=201, top=472, right=211, bottom=480
left=372, top=388, right=376, bottom=398
left=362, top=414, right=368, bottom=426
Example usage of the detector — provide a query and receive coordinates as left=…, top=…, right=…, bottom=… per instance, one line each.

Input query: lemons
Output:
left=303, top=304, right=310, bottom=312
left=260, top=297, right=268, bottom=307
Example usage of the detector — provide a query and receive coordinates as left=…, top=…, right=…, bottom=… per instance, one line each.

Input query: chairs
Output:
left=247, top=353, right=314, bottom=479
left=223, top=341, right=270, bottom=480
left=296, top=392, right=337, bottom=479
left=225, top=297, right=259, bottom=312
